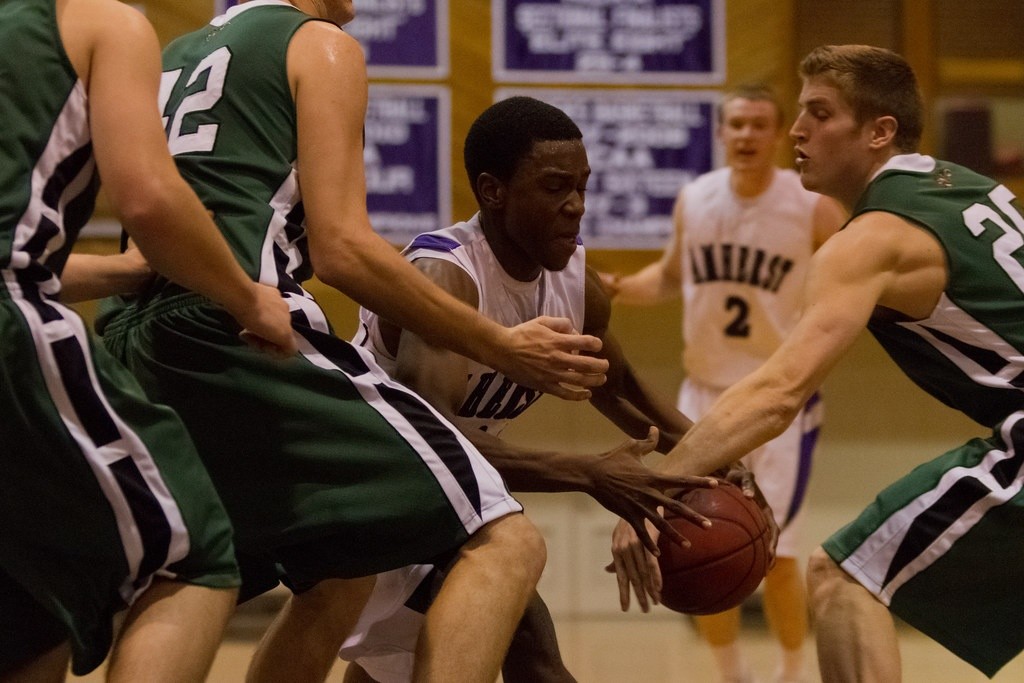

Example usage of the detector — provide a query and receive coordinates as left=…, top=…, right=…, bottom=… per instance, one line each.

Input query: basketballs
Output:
left=654, top=474, right=773, bottom=617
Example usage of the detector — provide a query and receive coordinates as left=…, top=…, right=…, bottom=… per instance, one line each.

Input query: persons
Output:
left=0, top=0, right=1024, bottom=683
